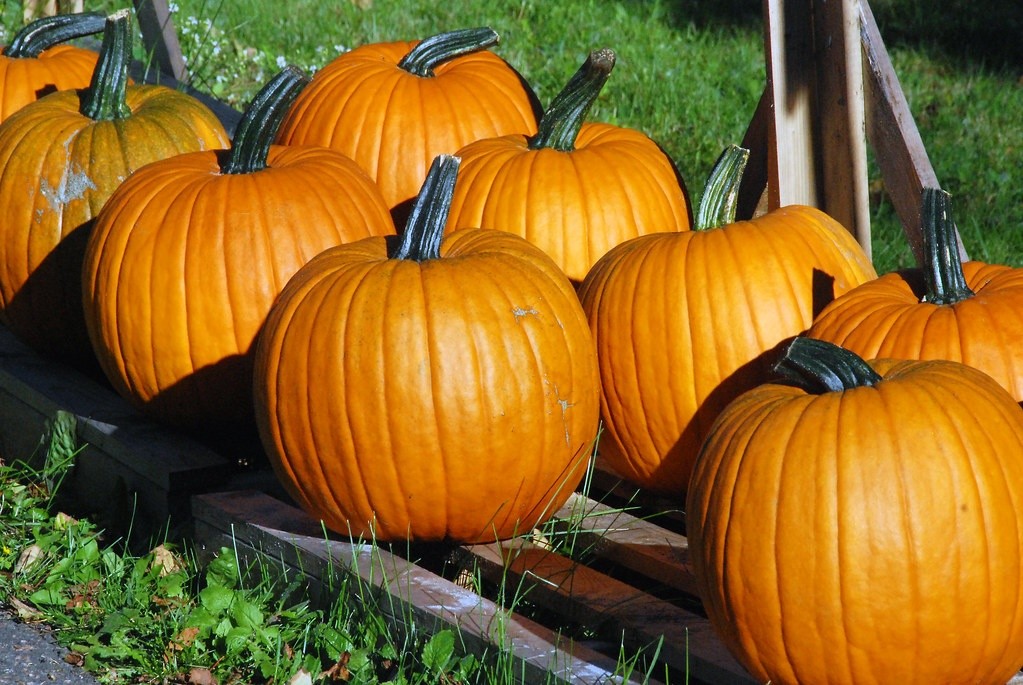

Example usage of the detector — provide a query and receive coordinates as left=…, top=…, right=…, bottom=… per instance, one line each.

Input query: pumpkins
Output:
left=0, top=11, right=1023, bottom=685
